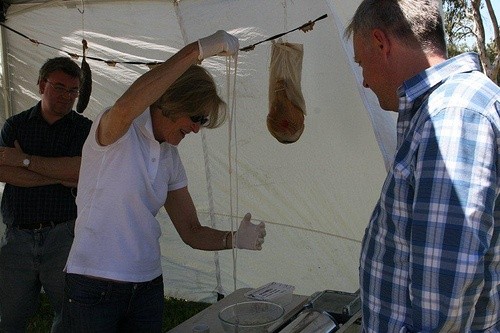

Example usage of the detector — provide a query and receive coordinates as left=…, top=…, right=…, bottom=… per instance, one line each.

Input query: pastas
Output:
left=225, top=53, right=275, bottom=333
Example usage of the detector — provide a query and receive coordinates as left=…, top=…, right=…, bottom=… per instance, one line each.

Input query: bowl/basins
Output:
left=244, top=282, right=295, bottom=307
left=218, top=301, right=285, bottom=333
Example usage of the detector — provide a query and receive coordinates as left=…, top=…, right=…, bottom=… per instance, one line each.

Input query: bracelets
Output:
left=223, top=231, right=231, bottom=248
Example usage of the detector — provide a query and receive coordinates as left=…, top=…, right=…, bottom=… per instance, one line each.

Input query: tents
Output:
left=0, top=0, right=398, bottom=304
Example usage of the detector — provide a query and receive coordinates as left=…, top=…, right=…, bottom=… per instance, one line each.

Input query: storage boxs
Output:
left=243, top=281, right=296, bottom=307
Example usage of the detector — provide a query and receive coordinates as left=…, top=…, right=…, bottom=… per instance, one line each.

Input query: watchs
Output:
left=22, top=155, right=32, bottom=168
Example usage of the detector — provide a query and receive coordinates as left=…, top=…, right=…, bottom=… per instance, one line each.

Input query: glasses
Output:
left=45, top=81, right=80, bottom=98
left=184, top=109, right=209, bottom=126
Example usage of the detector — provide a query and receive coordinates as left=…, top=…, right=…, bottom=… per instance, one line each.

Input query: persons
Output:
left=342, top=0, right=500, bottom=333
left=0, top=57, right=93, bottom=333
left=62, top=30, right=266, bottom=333
